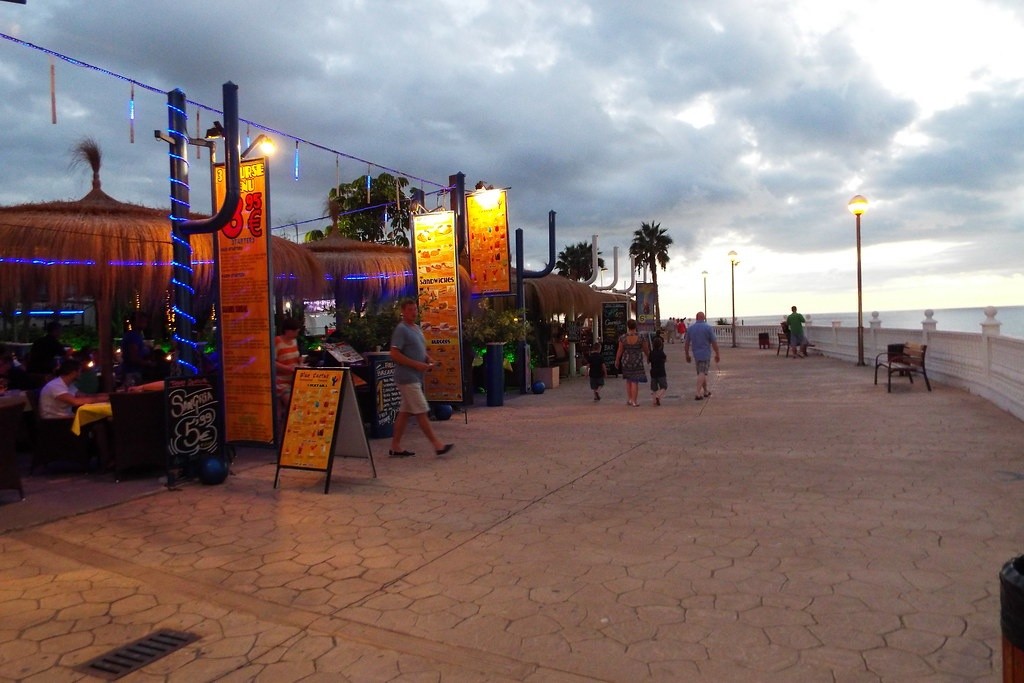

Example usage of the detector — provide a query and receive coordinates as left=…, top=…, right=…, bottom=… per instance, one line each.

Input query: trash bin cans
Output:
left=1001, top=555, right=1024, bottom=683
left=759, top=333, right=770, bottom=349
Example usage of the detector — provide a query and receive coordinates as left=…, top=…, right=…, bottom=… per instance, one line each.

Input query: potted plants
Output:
left=533, top=352, right=559, bottom=388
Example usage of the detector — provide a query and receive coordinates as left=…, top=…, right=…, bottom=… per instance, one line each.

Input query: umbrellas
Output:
left=0, top=138, right=639, bottom=321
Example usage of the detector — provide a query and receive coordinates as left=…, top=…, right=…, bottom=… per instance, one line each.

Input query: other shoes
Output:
left=681, top=339, right=684, bottom=343
left=695, top=395, right=703, bottom=400
left=807, top=342, right=814, bottom=347
left=627, top=399, right=632, bottom=405
left=594, top=392, right=601, bottom=400
left=803, top=351, right=808, bottom=356
left=703, top=391, right=711, bottom=397
left=389, top=449, right=416, bottom=456
left=632, top=401, right=640, bottom=407
left=793, top=355, right=796, bottom=358
left=798, top=352, right=804, bottom=358
left=437, top=443, right=454, bottom=455
left=652, top=396, right=660, bottom=406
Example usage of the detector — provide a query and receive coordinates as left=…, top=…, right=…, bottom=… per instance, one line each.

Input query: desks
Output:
left=71, top=403, right=116, bottom=471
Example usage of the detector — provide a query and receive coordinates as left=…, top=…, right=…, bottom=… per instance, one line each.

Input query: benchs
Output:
left=777, top=333, right=802, bottom=357
left=875, top=341, right=931, bottom=392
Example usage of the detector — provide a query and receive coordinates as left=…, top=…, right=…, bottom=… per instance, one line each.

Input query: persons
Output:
left=0, top=318, right=172, bottom=471
left=584, top=342, right=607, bottom=401
left=388, top=298, right=455, bottom=456
left=666, top=317, right=692, bottom=344
left=684, top=312, right=720, bottom=400
left=615, top=319, right=668, bottom=408
left=783, top=306, right=815, bottom=358
left=273, top=317, right=304, bottom=446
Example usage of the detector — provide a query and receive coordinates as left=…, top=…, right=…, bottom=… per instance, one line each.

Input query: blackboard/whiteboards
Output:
left=600, top=302, right=630, bottom=375
left=374, top=359, right=401, bottom=428
left=167, top=376, right=228, bottom=471
